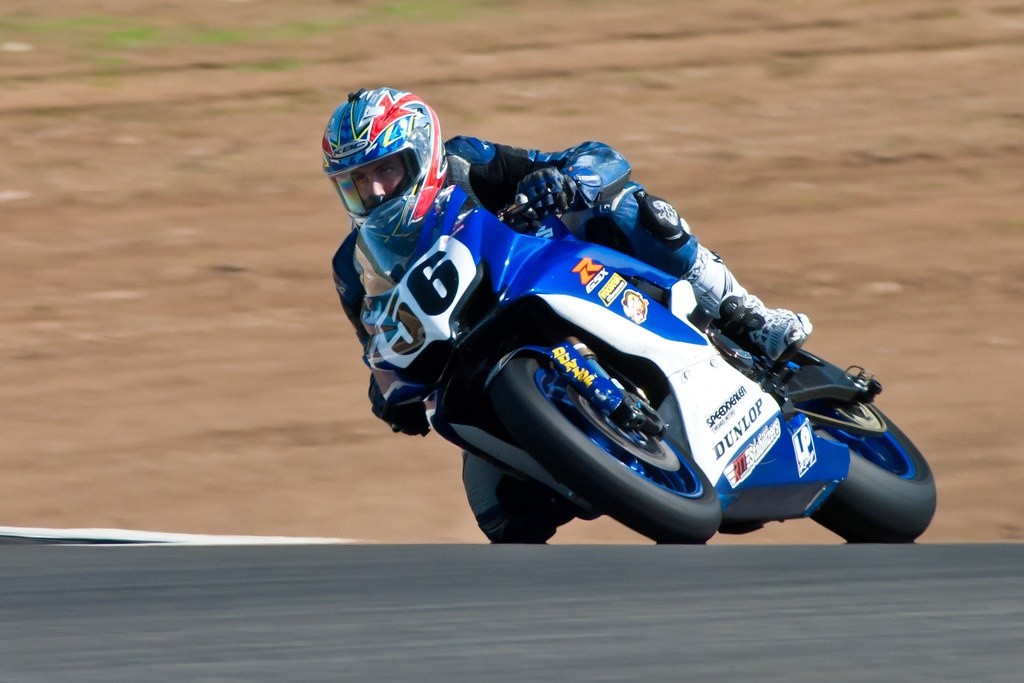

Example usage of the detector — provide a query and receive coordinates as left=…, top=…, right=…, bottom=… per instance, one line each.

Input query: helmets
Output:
left=320, top=87, right=449, bottom=247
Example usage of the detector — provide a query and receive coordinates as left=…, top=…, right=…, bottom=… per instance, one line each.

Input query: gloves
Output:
left=365, top=380, right=433, bottom=438
left=516, top=168, right=593, bottom=220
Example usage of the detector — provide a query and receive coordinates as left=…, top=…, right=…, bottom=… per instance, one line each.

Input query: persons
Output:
left=322, top=86, right=815, bottom=545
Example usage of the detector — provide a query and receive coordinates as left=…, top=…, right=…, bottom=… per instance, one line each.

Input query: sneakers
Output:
left=707, top=292, right=812, bottom=365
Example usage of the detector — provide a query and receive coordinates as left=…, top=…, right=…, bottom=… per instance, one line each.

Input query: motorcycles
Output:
left=352, top=196, right=936, bottom=544
